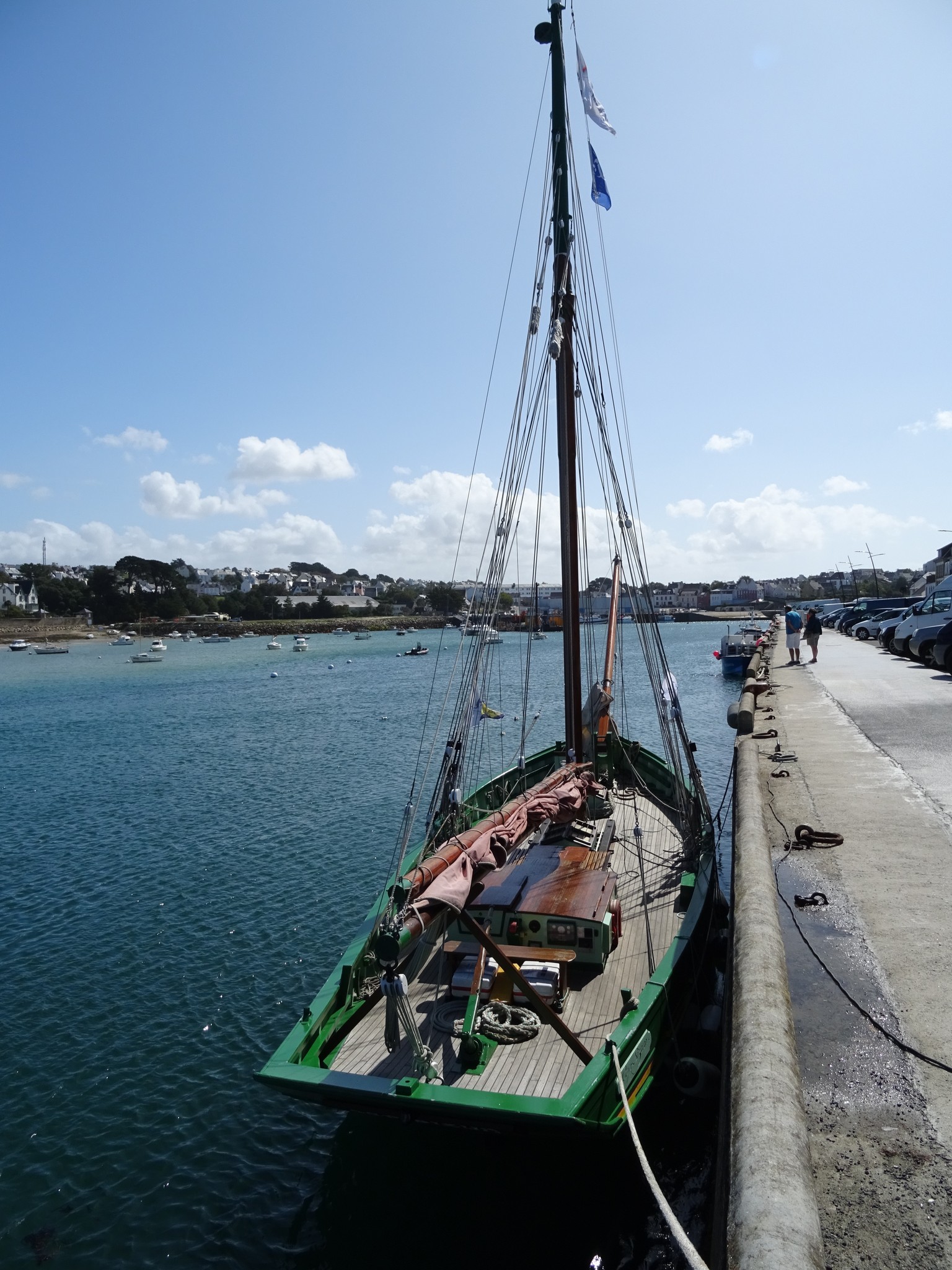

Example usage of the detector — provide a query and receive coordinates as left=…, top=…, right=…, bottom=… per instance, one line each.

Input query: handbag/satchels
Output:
left=803, top=631, right=811, bottom=639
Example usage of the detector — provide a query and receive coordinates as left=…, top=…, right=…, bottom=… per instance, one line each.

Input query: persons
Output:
left=784, top=604, right=804, bottom=665
left=804, top=608, right=822, bottom=663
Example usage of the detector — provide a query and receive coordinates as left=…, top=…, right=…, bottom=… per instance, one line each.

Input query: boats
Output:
left=130, top=652, right=164, bottom=662
left=405, top=642, right=429, bottom=655
left=462, top=625, right=497, bottom=637
left=294, top=635, right=310, bottom=640
left=407, top=627, right=418, bottom=633
left=445, top=623, right=457, bottom=630
left=9, top=638, right=33, bottom=651
left=168, top=630, right=197, bottom=642
left=532, top=632, right=546, bottom=640
left=202, top=634, right=231, bottom=643
left=459, top=624, right=466, bottom=631
left=483, top=637, right=504, bottom=644
left=396, top=628, right=406, bottom=635
left=294, top=638, right=308, bottom=651
left=111, top=636, right=133, bottom=646
left=250, top=723, right=715, bottom=1138
left=720, top=624, right=766, bottom=678
left=267, top=642, right=282, bottom=648
left=333, top=627, right=350, bottom=634
left=151, top=641, right=168, bottom=652
left=34, top=647, right=68, bottom=655
left=354, top=635, right=370, bottom=639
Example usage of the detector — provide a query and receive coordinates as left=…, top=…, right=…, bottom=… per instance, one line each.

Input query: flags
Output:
left=576, top=43, right=617, bottom=138
left=588, top=142, right=611, bottom=211
left=660, top=671, right=679, bottom=701
left=461, top=680, right=505, bottom=729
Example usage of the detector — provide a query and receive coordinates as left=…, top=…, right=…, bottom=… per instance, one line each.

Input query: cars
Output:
left=783, top=574, right=952, bottom=670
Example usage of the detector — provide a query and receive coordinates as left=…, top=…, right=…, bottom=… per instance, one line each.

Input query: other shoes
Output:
left=785, top=660, right=800, bottom=665
left=808, top=658, right=817, bottom=662
